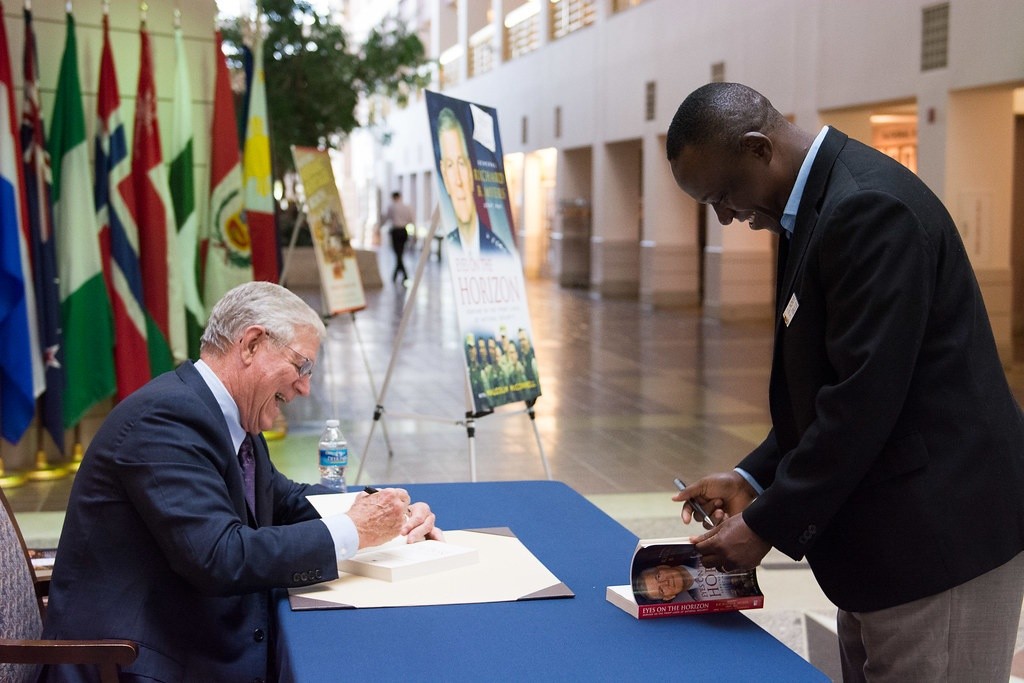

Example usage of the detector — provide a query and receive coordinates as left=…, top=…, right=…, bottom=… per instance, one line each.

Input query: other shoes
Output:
left=392, top=271, right=397, bottom=281
left=401, top=276, right=408, bottom=285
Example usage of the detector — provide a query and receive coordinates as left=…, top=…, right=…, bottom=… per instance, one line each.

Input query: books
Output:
left=305, top=489, right=456, bottom=582
left=605, top=536, right=763, bottom=620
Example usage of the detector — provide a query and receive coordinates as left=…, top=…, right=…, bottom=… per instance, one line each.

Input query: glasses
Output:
left=239, top=328, right=314, bottom=378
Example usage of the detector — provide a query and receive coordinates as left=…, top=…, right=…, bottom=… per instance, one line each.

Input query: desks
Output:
left=269, top=480, right=835, bottom=683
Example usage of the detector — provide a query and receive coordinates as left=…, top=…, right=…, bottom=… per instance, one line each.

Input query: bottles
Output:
left=318, top=419, right=349, bottom=494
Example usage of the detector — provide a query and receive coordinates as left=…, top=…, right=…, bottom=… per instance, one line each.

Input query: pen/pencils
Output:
left=364, top=487, right=412, bottom=517
left=673, top=478, right=714, bottom=527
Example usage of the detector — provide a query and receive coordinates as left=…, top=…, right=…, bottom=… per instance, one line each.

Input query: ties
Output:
left=240, top=433, right=257, bottom=530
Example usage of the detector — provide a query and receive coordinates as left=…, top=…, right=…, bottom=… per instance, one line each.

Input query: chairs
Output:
left=0, top=487, right=139, bottom=683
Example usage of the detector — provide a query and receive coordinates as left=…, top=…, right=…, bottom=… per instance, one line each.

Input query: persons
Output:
left=636, top=556, right=702, bottom=602
left=467, top=325, right=540, bottom=412
left=667, top=82, right=1024, bottom=683
left=437, top=108, right=512, bottom=259
left=379, top=192, right=415, bottom=284
left=31, top=281, right=442, bottom=683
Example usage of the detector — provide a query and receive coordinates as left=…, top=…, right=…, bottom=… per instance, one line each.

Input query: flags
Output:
left=0, top=0, right=284, bottom=460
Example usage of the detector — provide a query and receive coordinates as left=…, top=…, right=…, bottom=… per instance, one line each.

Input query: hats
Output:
left=466, top=333, right=476, bottom=347
left=500, top=324, right=508, bottom=336
